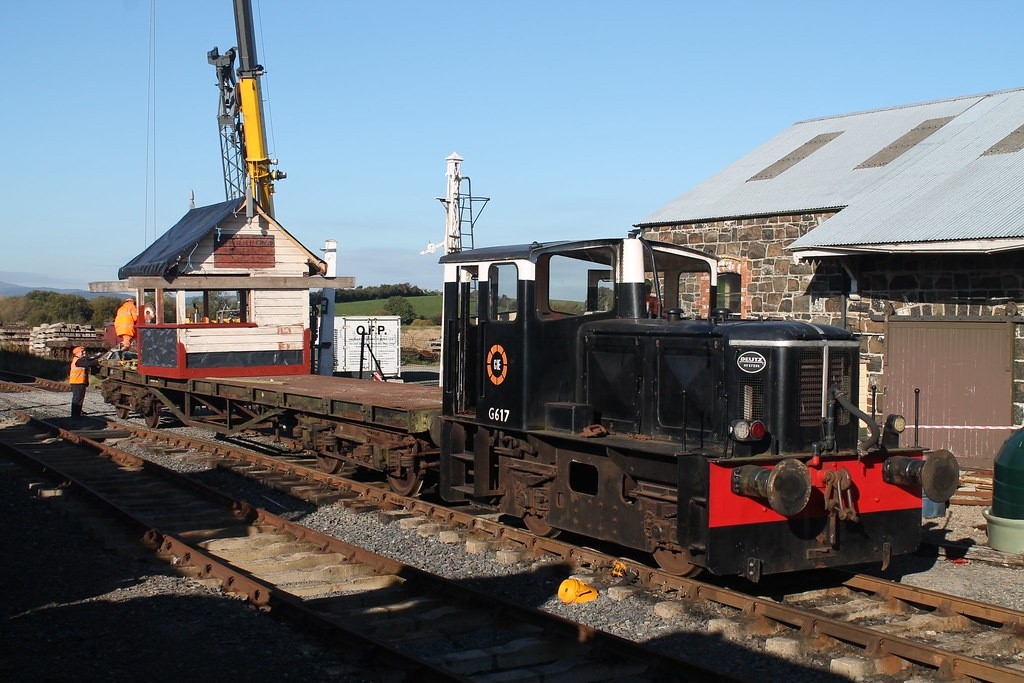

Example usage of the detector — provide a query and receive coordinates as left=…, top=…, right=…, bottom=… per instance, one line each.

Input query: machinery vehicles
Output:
left=207, top=0, right=287, bottom=223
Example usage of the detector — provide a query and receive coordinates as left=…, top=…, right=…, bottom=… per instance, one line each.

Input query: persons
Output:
left=645, top=279, right=664, bottom=316
left=69, top=347, right=102, bottom=425
left=114, top=299, right=138, bottom=361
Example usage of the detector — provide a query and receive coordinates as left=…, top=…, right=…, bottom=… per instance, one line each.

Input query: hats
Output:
left=125, top=299, right=133, bottom=302
left=73, top=347, right=85, bottom=354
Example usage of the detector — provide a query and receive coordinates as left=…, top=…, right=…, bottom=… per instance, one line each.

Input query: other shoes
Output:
left=72, top=424, right=87, bottom=430
left=123, top=354, right=132, bottom=361
left=115, top=351, right=121, bottom=361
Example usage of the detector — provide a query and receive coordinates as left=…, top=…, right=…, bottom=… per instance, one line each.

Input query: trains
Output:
left=96, top=228, right=961, bottom=580
